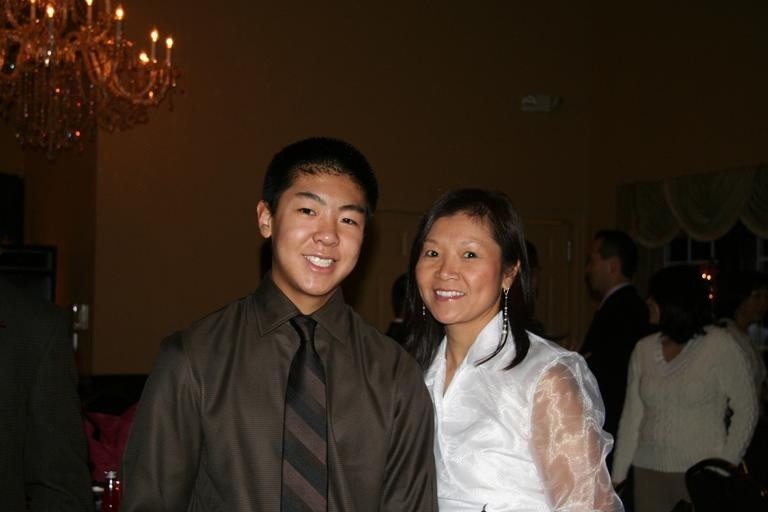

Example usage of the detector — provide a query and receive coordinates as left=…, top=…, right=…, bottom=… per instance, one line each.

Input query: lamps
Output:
left=0, top=1, right=185, bottom=155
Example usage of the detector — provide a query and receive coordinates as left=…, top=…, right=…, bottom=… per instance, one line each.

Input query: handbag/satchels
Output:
left=683, top=456, right=766, bottom=511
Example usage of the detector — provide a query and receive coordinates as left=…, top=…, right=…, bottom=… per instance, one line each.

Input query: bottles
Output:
left=101, top=471, right=120, bottom=512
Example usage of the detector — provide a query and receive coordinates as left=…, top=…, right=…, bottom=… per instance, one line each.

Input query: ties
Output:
left=280, top=316, right=328, bottom=511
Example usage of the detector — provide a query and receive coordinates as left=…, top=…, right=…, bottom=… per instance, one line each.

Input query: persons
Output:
left=117, top=133, right=438, bottom=510
left=384, top=272, right=417, bottom=346
left=612, top=262, right=764, bottom=512
left=525, top=240, right=545, bottom=336
left=404, top=186, right=627, bottom=511
left=579, top=227, right=656, bottom=475
left=713, top=265, right=768, bottom=512
left=0, top=272, right=98, bottom=510
